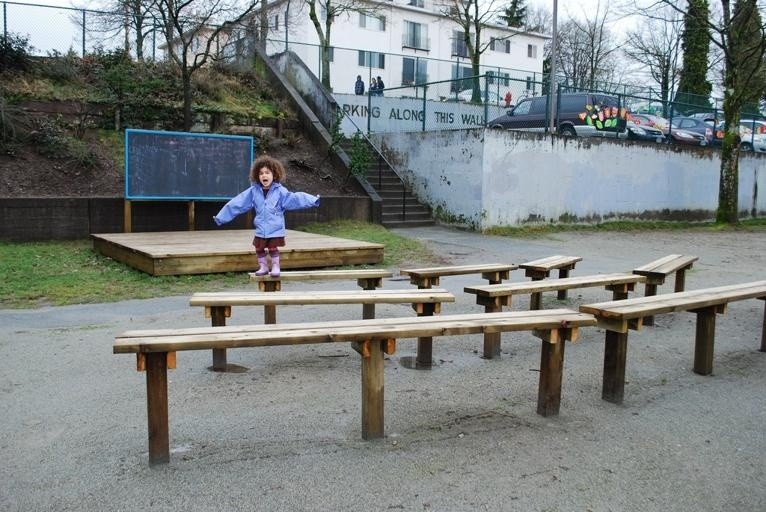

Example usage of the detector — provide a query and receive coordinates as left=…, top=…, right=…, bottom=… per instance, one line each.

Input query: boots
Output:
left=269, top=255, right=280, bottom=277
left=255, top=255, right=269, bottom=276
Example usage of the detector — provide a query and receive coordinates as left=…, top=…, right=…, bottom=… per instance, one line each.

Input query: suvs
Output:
left=487, top=94, right=628, bottom=138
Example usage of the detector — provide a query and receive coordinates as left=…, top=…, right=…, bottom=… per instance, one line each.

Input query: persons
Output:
left=370, top=78, right=377, bottom=97
left=376, top=77, right=385, bottom=96
left=213, top=154, right=321, bottom=277
left=354, top=74, right=364, bottom=95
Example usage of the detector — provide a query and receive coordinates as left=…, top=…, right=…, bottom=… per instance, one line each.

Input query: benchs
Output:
left=739, top=333, right=749, bottom=334
left=578, top=282, right=752, bottom=405
left=188, top=286, right=456, bottom=371
left=664, top=290, right=683, bottom=300
left=112, top=305, right=598, bottom=467
left=399, top=261, right=521, bottom=288
left=463, top=273, right=672, bottom=360
left=634, top=252, right=700, bottom=327
left=518, top=252, right=611, bottom=309
left=247, top=267, right=396, bottom=324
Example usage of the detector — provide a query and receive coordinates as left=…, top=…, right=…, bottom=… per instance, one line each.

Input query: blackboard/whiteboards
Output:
left=125, top=129, right=253, bottom=201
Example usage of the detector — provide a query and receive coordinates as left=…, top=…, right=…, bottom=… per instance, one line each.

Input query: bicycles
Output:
left=516, top=90, right=538, bottom=105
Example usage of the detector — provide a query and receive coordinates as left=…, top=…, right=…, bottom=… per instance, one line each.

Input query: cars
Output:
left=627, top=111, right=766, bottom=153
left=444, top=88, right=504, bottom=105
